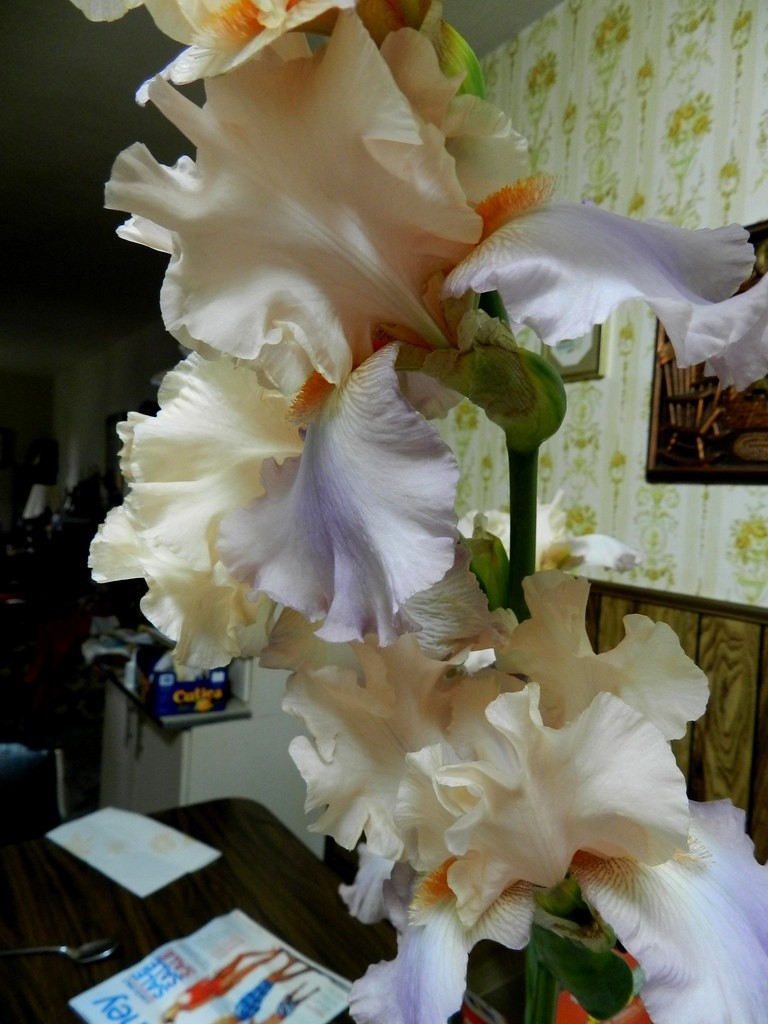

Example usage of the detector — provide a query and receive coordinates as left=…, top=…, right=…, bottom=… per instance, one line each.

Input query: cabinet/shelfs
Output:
left=96, top=653, right=329, bottom=861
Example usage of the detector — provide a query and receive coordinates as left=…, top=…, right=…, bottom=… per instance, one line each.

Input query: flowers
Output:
left=67, top=1, right=764, bottom=1024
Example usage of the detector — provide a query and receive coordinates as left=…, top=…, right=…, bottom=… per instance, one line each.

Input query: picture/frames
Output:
left=644, top=217, right=766, bottom=487
left=541, top=324, right=602, bottom=384
left=582, top=579, right=766, bottom=866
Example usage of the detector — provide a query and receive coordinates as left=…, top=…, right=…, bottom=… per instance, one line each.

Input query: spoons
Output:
left=0, top=935, right=119, bottom=963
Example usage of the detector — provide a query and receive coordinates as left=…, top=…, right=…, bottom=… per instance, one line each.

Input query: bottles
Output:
left=124, top=646, right=137, bottom=691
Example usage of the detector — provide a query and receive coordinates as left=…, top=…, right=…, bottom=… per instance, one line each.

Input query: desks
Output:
left=2, top=795, right=497, bottom=1022
